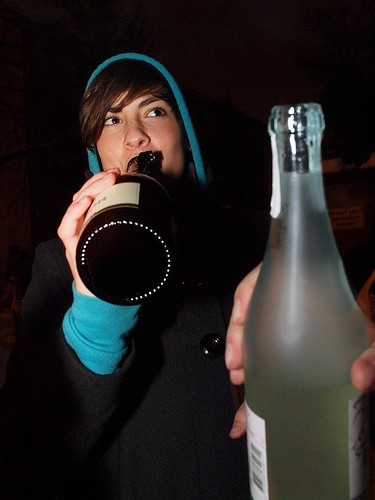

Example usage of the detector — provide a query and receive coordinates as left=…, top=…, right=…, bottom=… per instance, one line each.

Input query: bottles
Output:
left=243, top=103, right=373, bottom=500
left=76, top=150, right=179, bottom=304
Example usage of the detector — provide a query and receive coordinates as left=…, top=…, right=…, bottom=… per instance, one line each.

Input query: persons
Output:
left=5, top=51, right=270, bottom=500
left=224, top=245, right=375, bottom=440
left=7, top=244, right=37, bottom=298
